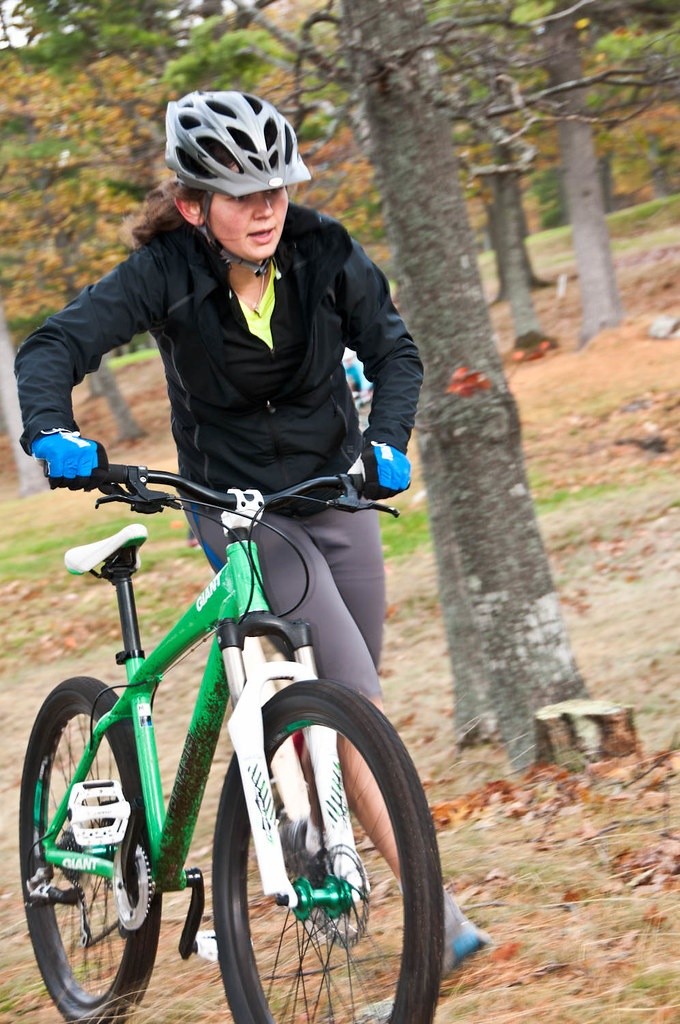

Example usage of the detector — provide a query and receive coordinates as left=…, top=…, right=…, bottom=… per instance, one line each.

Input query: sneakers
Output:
left=442, top=884, right=492, bottom=980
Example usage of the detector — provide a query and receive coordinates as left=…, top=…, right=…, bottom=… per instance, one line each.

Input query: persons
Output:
left=16, top=90, right=496, bottom=982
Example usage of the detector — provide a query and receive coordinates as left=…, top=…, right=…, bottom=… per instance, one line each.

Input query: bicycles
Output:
left=22, top=465, right=443, bottom=1024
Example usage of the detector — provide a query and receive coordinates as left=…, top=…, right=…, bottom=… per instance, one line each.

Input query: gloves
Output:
left=34, top=432, right=108, bottom=494
left=347, top=441, right=411, bottom=501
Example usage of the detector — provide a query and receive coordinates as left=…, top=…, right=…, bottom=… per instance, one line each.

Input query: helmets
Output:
left=164, top=90, right=312, bottom=199
